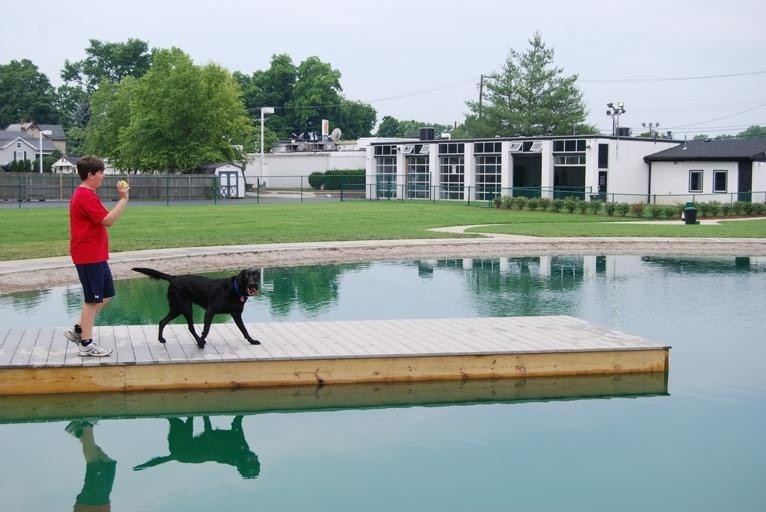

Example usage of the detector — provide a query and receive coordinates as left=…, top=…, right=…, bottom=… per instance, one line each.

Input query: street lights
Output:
left=606, top=102, right=627, bottom=136
left=260, top=106, right=276, bottom=182
left=642, top=122, right=661, bottom=138
left=38, top=130, right=53, bottom=174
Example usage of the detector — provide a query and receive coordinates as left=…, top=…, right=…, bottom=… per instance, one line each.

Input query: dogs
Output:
left=131, top=266, right=261, bottom=349
left=132, top=416, right=261, bottom=479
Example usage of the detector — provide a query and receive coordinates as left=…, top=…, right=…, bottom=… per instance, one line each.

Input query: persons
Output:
left=64, top=416, right=117, bottom=511
left=62, top=155, right=131, bottom=356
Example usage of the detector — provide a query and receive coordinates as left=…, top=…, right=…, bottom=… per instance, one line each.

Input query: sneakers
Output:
left=78, top=341, right=113, bottom=356
left=63, top=325, right=83, bottom=344
